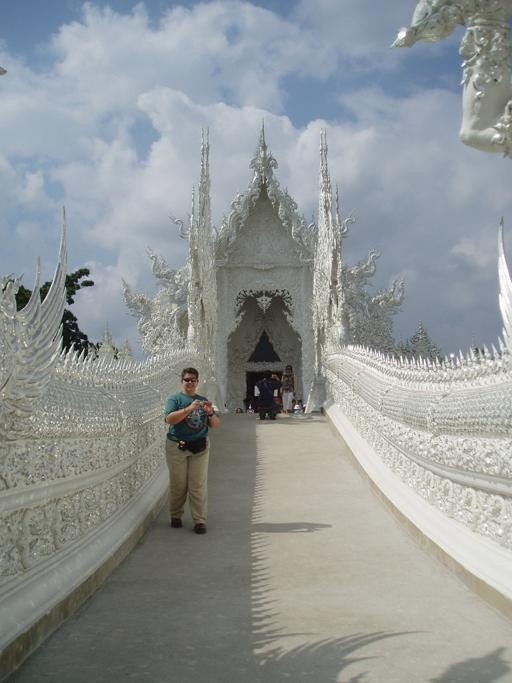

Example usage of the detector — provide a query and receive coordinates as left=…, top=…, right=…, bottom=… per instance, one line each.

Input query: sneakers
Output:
left=194, top=523, right=206, bottom=534
left=171, top=517, right=182, bottom=527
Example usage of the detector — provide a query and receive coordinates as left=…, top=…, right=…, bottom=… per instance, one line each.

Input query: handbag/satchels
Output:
left=185, top=437, right=207, bottom=454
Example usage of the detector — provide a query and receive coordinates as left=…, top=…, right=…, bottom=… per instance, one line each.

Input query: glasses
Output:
left=183, top=377, right=198, bottom=382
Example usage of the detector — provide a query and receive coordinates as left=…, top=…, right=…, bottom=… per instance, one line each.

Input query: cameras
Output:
left=196, top=402, right=205, bottom=407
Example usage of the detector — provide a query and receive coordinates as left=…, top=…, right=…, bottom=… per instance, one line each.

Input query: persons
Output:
left=164, top=368, right=219, bottom=534
left=235, top=365, right=307, bottom=419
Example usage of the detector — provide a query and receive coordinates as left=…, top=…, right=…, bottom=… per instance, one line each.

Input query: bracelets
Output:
left=207, top=411, right=216, bottom=417
left=183, top=408, right=187, bottom=414
